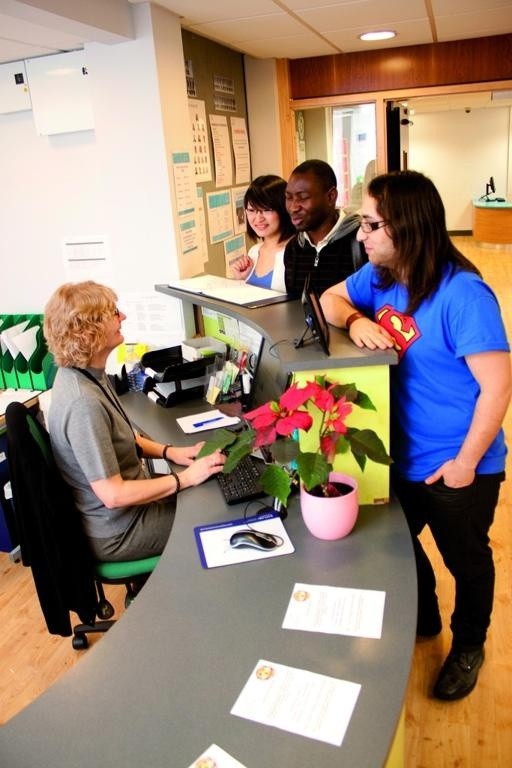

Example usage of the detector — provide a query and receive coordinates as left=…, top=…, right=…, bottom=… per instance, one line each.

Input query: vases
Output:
left=300, top=473, right=359, bottom=540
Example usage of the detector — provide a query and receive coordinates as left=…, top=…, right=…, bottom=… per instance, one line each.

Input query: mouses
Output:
left=231, top=530, right=277, bottom=551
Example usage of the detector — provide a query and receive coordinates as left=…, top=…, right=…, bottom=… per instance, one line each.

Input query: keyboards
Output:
left=496, top=198, right=505, bottom=202
left=215, top=450, right=271, bottom=505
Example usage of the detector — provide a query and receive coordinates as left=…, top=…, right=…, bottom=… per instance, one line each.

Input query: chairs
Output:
left=6, top=401, right=161, bottom=649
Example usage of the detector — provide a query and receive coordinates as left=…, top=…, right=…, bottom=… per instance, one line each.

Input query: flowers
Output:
left=197, top=373, right=394, bottom=507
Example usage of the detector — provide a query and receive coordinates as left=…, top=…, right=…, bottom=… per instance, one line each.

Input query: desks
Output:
left=470, top=196, right=511, bottom=244
left=0, top=384, right=40, bottom=429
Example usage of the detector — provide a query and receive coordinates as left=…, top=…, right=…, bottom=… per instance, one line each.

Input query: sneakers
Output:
left=432, top=651, right=485, bottom=700
left=418, top=609, right=442, bottom=637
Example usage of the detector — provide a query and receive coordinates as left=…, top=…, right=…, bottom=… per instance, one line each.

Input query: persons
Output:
left=229, top=174, right=296, bottom=293
left=318, top=169, right=512, bottom=699
left=283, top=159, right=368, bottom=301
left=42, top=280, right=227, bottom=595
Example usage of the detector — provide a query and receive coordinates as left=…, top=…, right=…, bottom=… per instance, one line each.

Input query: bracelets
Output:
left=162, top=443, right=172, bottom=459
left=344, top=312, right=365, bottom=330
left=170, top=472, right=180, bottom=495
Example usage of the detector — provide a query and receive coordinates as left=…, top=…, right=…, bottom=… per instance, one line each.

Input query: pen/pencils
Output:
left=193, top=417, right=225, bottom=427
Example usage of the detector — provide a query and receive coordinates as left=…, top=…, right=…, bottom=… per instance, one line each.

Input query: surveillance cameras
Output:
left=464, top=108, right=471, bottom=113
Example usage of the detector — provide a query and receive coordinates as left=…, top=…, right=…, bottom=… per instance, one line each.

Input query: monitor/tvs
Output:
left=243, top=337, right=292, bottom=463
left=486, top=176, right=495, bottom=202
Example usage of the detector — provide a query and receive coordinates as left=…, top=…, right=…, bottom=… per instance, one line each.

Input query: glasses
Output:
left=245, top=208, right=272, bottom=215
left=360, top=220, right=389, bottom=233
